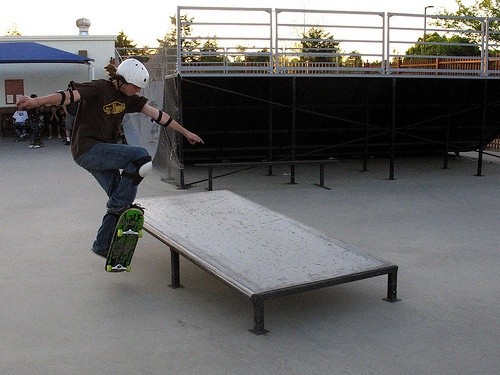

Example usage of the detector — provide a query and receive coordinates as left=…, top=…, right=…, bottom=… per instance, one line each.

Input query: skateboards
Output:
left=103, top=205, right=145, bottom=274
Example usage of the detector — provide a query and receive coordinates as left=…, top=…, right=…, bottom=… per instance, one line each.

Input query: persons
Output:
left=12, top=81, right=78, bottom=149
left=16, top=58, right=204, bottom=259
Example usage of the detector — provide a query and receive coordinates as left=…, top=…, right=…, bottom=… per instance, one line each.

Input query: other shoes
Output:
left=58, top=135, right=62, bottom=139
left=93, top=248, right=109, bottom=259
left=120, top=204, right=145, bottom=217
left=28, top=144, right=41, bottom=149
left=48, top=135, right=52, bottom=139
left=64, top=141, right=71, bottom=145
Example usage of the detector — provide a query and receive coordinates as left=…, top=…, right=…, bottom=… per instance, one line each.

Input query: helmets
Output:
left=116, top=58, right=151, bottom=90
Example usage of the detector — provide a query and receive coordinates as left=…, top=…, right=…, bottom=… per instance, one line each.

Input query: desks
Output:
left=122, top=189, right=398, bottom=335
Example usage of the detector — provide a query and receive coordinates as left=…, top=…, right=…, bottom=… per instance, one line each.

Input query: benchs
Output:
left=0, top=106, right=70, bottom=140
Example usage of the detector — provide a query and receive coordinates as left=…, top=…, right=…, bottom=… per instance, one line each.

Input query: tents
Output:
left=0, top=42, right=96, bottom=82
